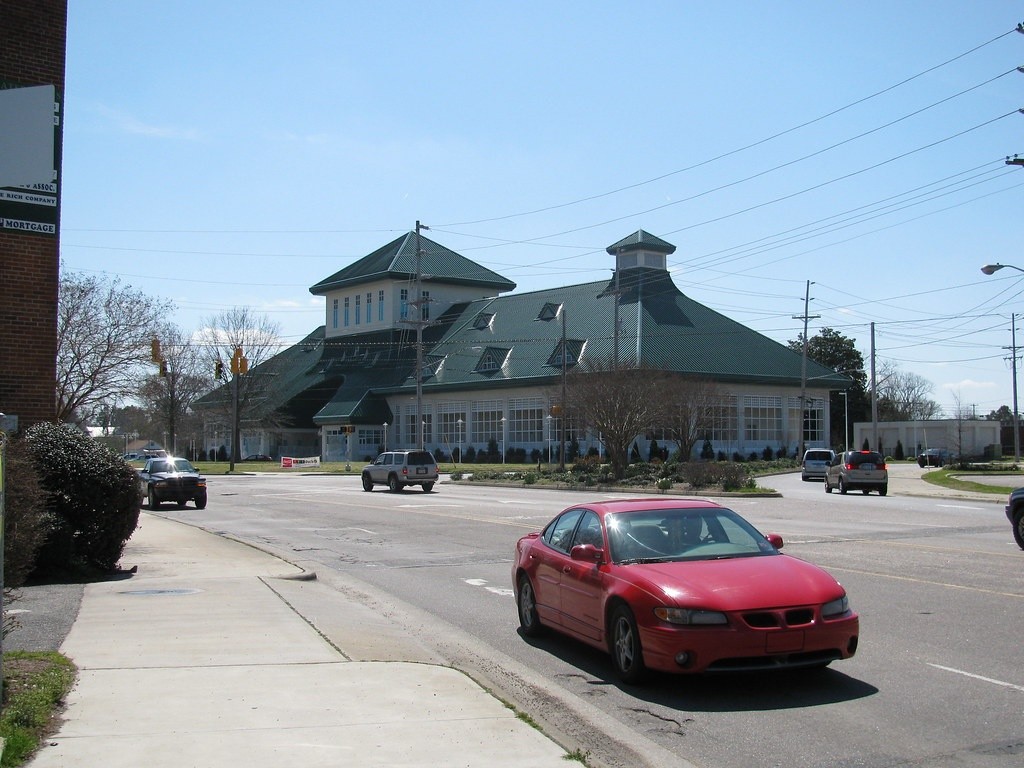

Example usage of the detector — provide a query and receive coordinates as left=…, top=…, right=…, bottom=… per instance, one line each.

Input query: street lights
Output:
left=838, top=391, right=849, bottom=453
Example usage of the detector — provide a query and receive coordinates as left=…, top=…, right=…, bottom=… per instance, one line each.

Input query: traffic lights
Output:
left=216, top=362, right=223, bottom=380
left=159, top=360, right=167, bottom=377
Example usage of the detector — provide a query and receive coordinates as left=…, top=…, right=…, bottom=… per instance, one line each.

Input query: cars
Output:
left=509, top=497, right=859, bottom=689
left=121, top=452, right=160, bottom=462
left=917, top=448, right=960, bottom=467
left=239, top=454, right=272, bottom=461
left=1005, top=487, right=1024, bottom=551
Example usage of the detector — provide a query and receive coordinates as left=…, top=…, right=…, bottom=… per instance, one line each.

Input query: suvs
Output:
left=824, top=450, right=889, bottom=496
left=135, top=457, right=207, bottom=509
left=361, top=449, right=440, bottom=492
left=801, top=448, right=837, bottom=481
